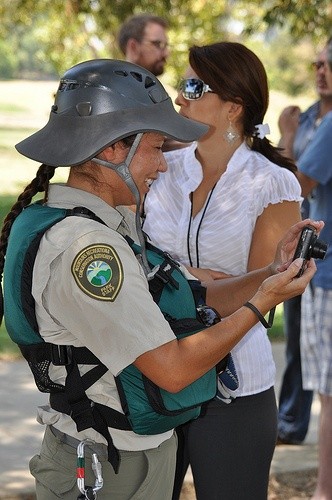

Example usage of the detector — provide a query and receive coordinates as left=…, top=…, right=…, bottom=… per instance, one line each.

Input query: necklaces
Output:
left=186, top=183, right=217, bottom=268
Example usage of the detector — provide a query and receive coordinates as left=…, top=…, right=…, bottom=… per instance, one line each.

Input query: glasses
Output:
left=178, top=78, right=213, bottom=100
left=138, top=41, right=167, bottom=49
left=196, top=305, right=228, bottom=373
left=311, top=60, right=332, bottom=71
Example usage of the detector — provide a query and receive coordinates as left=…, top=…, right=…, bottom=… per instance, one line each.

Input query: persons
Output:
left=277, top=39, right=332, bottom=500
left=0, top=59, right=325, bottom=500
left=142, top=42, right=305, bottom=500
left=120, top=14, right=169, bottom=75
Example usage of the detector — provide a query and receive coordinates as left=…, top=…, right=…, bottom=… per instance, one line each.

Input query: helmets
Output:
left=15, top=59, right=209, bottom=167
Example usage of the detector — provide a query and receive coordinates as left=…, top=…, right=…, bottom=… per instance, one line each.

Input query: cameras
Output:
left=292, top=224, right=328, bottom=277
